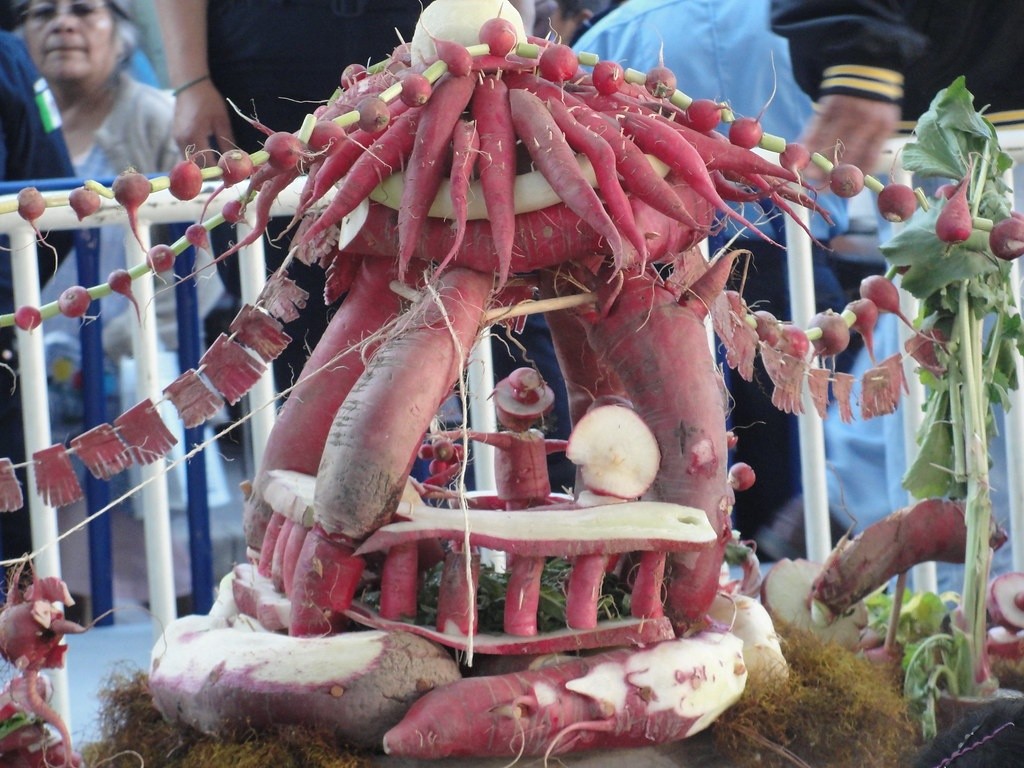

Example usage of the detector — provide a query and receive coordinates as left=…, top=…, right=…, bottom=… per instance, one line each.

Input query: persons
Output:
left=769, top=0, right=1023, bottom=607
left=158, top=0, right=579, bottom=546
left=0, top=0, right=264, bottom=589
left=535, top=0, right=852, bottom=565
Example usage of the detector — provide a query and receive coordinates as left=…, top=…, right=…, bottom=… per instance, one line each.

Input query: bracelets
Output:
left=172, top=74, right=210, bottom=96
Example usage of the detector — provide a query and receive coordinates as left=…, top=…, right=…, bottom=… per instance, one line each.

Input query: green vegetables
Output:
left=345, top=548, right=634, bottom=630
